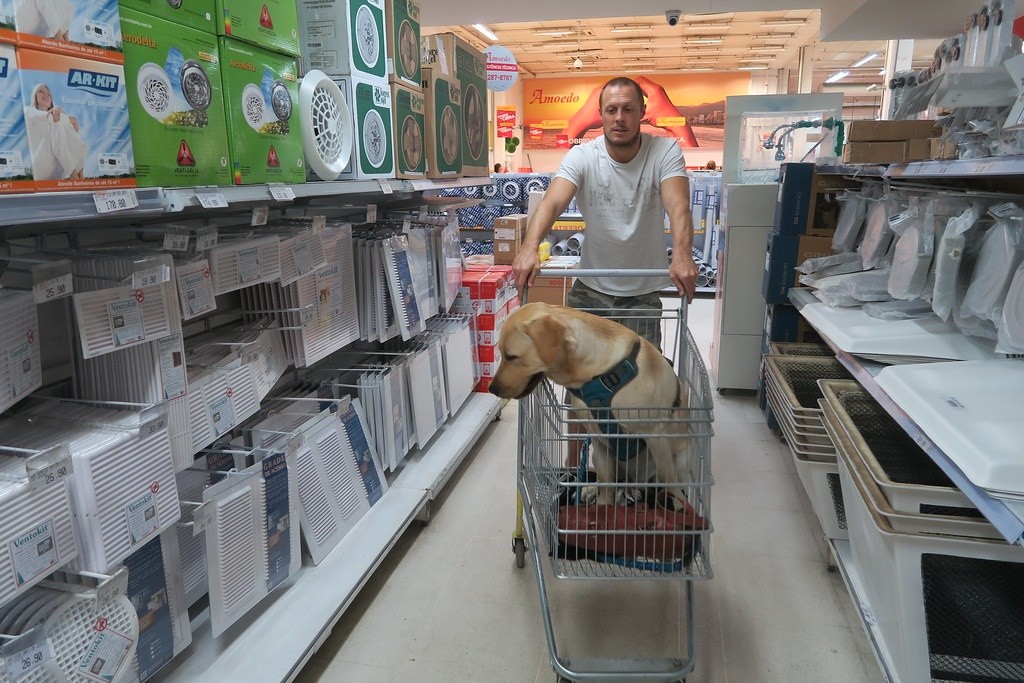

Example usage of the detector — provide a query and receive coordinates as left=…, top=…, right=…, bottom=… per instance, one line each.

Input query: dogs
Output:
left=488, top=301, right=691, bottom=508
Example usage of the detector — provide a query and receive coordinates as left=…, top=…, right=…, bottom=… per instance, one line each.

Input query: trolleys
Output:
left=511, top=269, right=716, bottom=682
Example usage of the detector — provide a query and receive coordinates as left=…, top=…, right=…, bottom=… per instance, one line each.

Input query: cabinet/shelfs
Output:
left=765, top=19, right=1024, bottom=683
left=0, top=2, right=504, bottom=683
left=461, top=219, right=718, bottom=292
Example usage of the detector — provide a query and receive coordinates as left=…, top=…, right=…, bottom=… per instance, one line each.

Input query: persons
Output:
left=707, top=161, right=716, bottom=170
left=511, top=77, right=698, bottom=465
left=23, top=83, right=85, bottom=180
left=494, top=164, right=503, bottom=174
left=14, top=0, right=73, bottom=41
left=562, top=76, right=699, bottom=147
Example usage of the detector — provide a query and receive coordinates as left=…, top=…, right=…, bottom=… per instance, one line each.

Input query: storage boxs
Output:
left=760, top=341, right=1024, bottom=683
left=847, top=120, right=936, bottom=141
left=452, top=213, right=582, bottom=391
left=841, top=139, right=930, bottom=162
left=0, top=0, right=490, bottom=186
left=930, top=127, right=958, bottom=159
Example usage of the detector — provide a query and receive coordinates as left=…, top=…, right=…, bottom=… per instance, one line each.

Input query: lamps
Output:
left=848, top=51, right=878, bottom=67
left=825, top=71, right=850, bottom=85
left=472, top=24, right=498, bottom=40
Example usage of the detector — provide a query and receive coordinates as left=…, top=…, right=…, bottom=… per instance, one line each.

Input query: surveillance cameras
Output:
left=664, top=9, right=681, bottom=26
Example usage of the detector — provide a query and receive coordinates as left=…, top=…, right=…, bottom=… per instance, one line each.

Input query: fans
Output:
left=298, top=68, right=353, bottom=181
